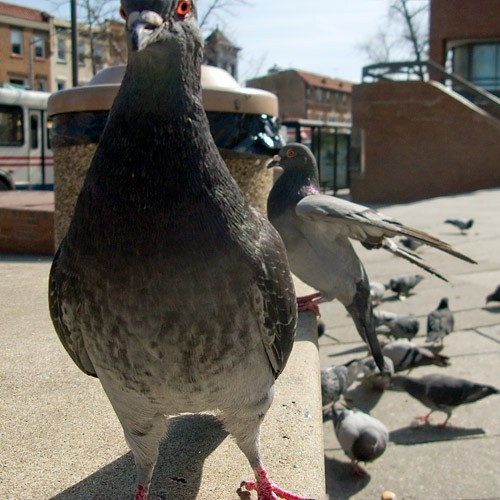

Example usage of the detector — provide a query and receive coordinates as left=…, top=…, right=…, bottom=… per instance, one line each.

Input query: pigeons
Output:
left=388, top=274, right=425, bottom=302
left=444, top=219, right=474, bottom=235
left=50, top=0, right=309, bottom=500
left=317, top=285, right=500, bottom=422
left=385, top=374, right=500, bottom=428
left=267, top=143, right=479, bottom=375
left=331, top=403, right=390, bottom=477
left=399, top=238, right=424, bottom=252
left=367, top=282, right=385, bottom=306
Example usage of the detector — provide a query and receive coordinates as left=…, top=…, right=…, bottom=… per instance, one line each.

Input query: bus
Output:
left=0, top=85, right=55, bottom=192
